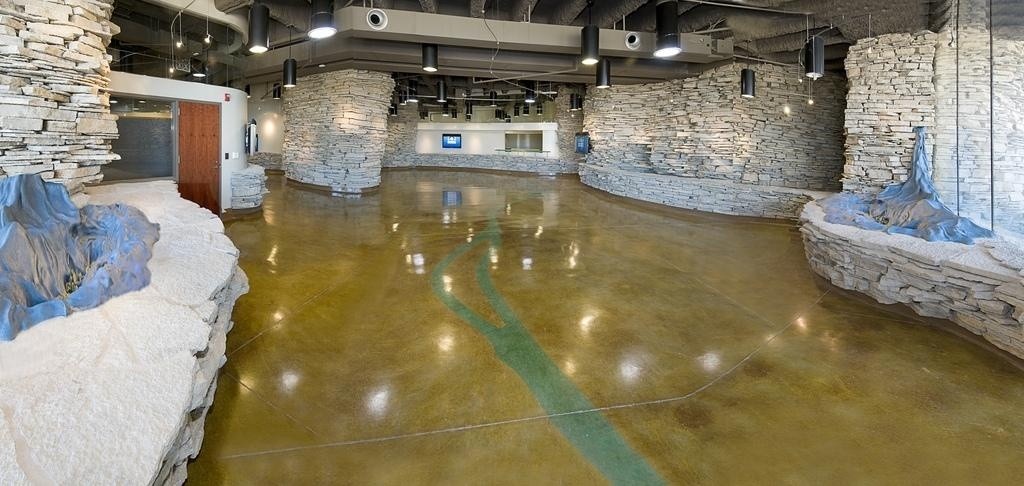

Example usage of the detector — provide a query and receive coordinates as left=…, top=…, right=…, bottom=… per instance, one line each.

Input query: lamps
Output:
left=595, top=56, right=611, bottom=88
left=654, top=0, right=683, bottom=58
left=390, top=72, right=584, bottom=125
left=740, top=39, right=756, bottom=99
left=169, top=0, right=337, bottom=100
left=580, top=0, right=600, bottom=65
left=805, top=16, right=825, bottom=80
left=422, top=43, right=438, bottom=72
